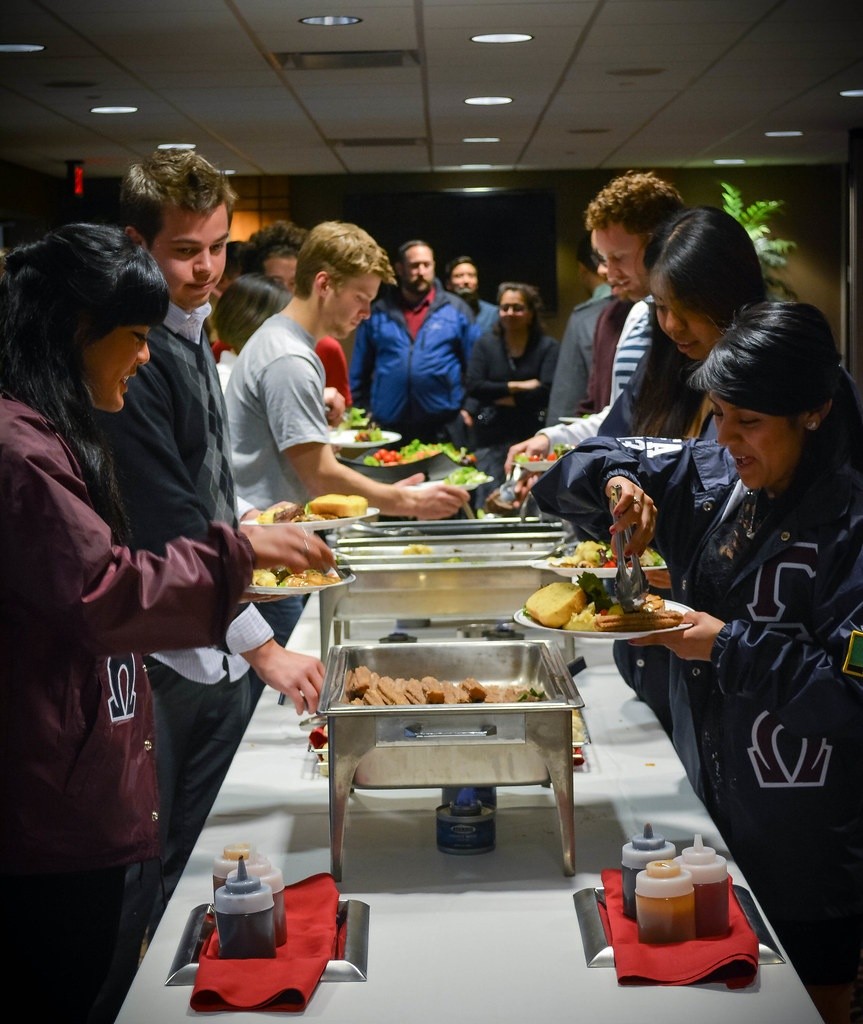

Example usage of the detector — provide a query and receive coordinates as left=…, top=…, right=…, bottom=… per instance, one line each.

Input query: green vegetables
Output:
left=335, top=408, right=388, bottom=441
left=363, top=455, right=381, bottom=466
left=273, top=555, right=343, bottom=588
left=514, top=455, right=529, bottom=463
left=577, top=571, right=614, bottom=609
left=554, top=443, right=572, bottom=459
left=596, top=540, right=664, bottom=566
left=396, top=440, right=486, bottom=485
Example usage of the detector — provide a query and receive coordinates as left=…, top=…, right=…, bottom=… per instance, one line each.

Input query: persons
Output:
left=598, top=206, right=860, bottom=802
left=545, top=234, right=612, bottom=428
left=460, top=282, right=561, bottom=512
left=96, top=147, right=327, bottom=948
left=212, top=225, right=353, bottom=409
left=529, top=300, right=863, bottom=1024
left=211, top=273, right=347, bottom=455
left=0, top=223, right=337, bottom=1024
left=573, top=229, right=635, bottom=418
left=444, top=257, right=499, bottom=338
left=350, top=241, right=482, bottom=453
left=224, top=221, right=471, bottom=722
left=505, top=172, right=686, bottom=496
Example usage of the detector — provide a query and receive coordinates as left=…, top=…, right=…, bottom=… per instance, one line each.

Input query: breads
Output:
left=526, top=582, right=586, bottom=628
left=307, top=494, right=368, bottom=518
left=251, top=567, right=280, bottom=588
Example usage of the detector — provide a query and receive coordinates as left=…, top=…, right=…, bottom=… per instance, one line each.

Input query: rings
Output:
left=634, top=497, right=642, bottom=509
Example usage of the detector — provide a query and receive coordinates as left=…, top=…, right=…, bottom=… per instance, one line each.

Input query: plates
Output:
left=328, top=430, right=402, bottom=448
left=513, top=598, right=697, bottom=642
left=529, top=557, right=667, bottom=579
left=407, top=475, right=494, bottom=490
left=559, top=416, right=587, bottom=424
left=247, top=574, right=355, bottom=595
left=337, top=447, right=445, bottom=478
left=242, top=508, right=381, bottom=531
left=510, top=461, right=556, bottom=471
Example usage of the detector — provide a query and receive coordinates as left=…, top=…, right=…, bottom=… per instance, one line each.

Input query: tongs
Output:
left=610, top=483, right=651, bottom=614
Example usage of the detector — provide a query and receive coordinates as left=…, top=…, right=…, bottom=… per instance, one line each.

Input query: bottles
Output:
left=213, top=842, right=289, bottom=958
left=621, top=821, right=727, bottom=944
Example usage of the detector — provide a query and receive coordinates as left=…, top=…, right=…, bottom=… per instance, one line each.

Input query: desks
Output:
left=115, top=584, right=827, bottom=1024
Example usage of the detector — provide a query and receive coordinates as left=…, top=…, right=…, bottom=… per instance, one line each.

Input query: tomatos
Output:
left=530, top=452, right=556, bottom=462
left=373, top=448, right=401, bottom=463
